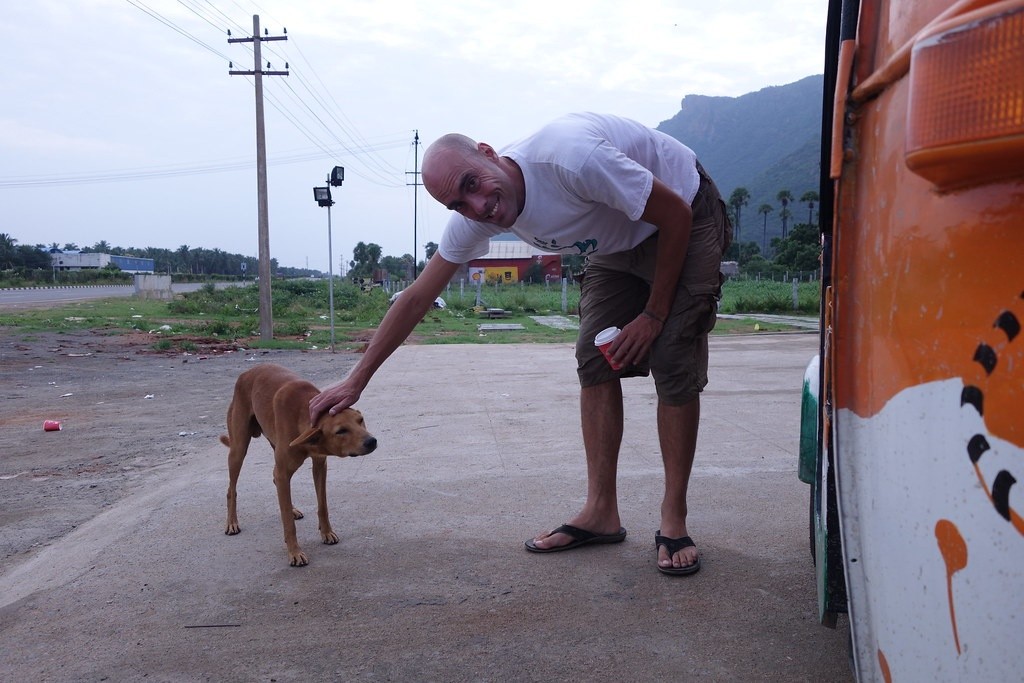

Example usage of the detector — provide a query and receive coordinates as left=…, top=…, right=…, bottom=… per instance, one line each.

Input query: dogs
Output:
left=220, top=363, right=378, bottom=568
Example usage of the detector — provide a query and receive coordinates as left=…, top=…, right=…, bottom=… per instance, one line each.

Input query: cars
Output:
left=795, top=0, right=1022, bottom=683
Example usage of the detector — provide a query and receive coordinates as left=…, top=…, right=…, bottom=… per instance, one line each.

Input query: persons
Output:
left=304, top=108, right=736, bottom=580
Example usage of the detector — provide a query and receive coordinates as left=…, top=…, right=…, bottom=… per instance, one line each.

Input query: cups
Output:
left=594, top=326, right=625, bottom=370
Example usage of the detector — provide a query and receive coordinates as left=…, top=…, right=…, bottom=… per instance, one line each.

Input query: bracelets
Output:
left=641, top=306, right=665, bottom=325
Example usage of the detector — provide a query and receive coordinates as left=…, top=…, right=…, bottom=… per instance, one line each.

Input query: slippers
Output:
left=654, top=530, right=701, bottom=575
left=524, top=524, right=627, bottom=552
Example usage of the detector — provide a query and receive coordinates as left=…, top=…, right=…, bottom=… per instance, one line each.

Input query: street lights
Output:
left=314, top=166, right=345, bottom=351
